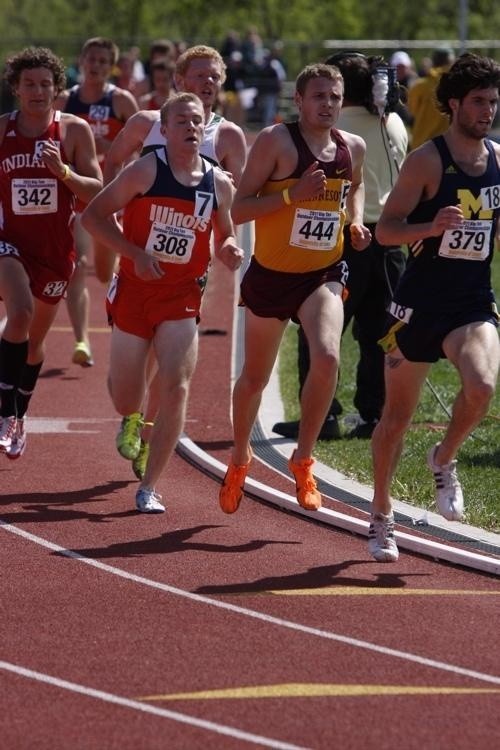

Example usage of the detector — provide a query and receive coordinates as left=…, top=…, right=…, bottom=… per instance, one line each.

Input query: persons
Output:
left=0, top=46, right=105, bottom=458
left=105, top=45, right=248, bottom=481
left=1, top=40, right=498, bottom=150
left=272, top=49, right=410, bottom=438
left=53, top=38, right=139, bottom=368
left=217, top=63, right=372, bottom=515
left=79, top=91, right=245, bottom=512
left=368, top=53, right=500, bottom=561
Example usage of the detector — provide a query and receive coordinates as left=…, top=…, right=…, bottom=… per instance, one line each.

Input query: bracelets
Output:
left=61, top=164, right=70, bottom=182
left=283, top=188, right=289, bottom=207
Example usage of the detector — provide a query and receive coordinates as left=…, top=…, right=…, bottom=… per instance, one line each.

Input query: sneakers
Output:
left=287, top=447, right=322, bottom=511
left=343, top=420, right=381, bottom=440
left=0, top=414, right=18, bottom=455
left=135, top=484, right=166, bottom=514
left=116, top=411, right=146, bottom=461
left=72, top=339, right=94, bottom=368
left=5, top=412, right=27, bottom=460
left=132, top=438, right=151, bottom=481
left=272, top=411, right=341, bottom=441
left=426, top=440, right=464, bottom=521
left=218, top=442, right=254, bottom=514
left=367, top=497, right=400, bottom=562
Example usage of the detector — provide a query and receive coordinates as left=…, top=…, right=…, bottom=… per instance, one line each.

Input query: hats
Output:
left=391, top=50, right=412, bottom=69
